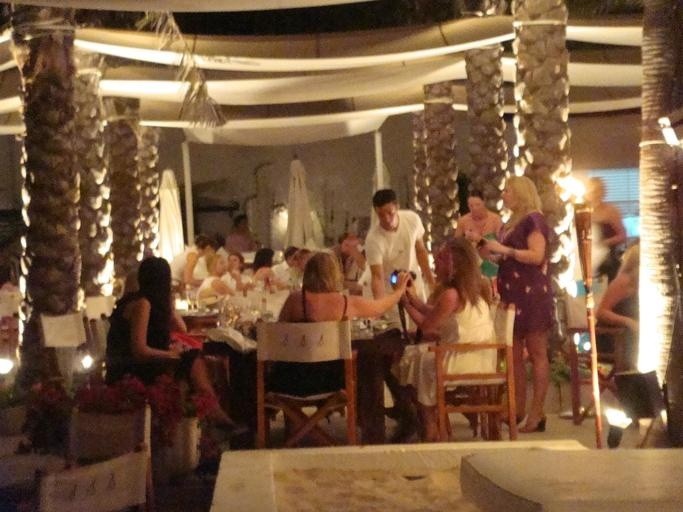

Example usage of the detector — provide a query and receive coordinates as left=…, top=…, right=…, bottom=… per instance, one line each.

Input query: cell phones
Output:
left=391, top=269, right=417, bottom=289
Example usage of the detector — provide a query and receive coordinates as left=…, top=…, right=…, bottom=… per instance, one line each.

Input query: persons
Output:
left=168, top=233, right=300, bottom=312
left=1, top=261, right=25, bottom=321
left=99, top=256, right=249, bottom=482
left=267, top=250, right=411, bottom=419
left=337, top=233, right=366, bottom=282
left=597, top=239, right=638, bottom=384
left=271, top=247, right=312, bottom=287
left=381, top=231, right=499, bottom=442
left=469, top=174, right=560, bottom=434
left=365, top=188, right=436, bottom=332
left=450, top=188, right=507, bottom=279
left=579, top=173, right=626, bottom=280
left=224, top=214, right=257, bottom=251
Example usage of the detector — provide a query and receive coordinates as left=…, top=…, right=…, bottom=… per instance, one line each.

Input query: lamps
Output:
left=614, top=369, right=674, bottom=445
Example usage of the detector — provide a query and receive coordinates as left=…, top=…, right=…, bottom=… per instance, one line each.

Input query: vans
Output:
left=518, top=411, right=546, bottom=432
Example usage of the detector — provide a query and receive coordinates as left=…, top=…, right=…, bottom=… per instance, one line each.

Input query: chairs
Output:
left=236, top=269, right=638, bottom=446
left=0, top=268, right=235, bottom=510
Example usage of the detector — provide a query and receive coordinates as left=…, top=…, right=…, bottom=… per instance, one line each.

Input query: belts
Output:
left=227, top=305, right=240, bottom=325
left=187, top=289, right=198, bottom=313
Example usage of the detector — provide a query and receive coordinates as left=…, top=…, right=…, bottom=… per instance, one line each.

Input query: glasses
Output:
left=614, top=369, right=674, bottom=445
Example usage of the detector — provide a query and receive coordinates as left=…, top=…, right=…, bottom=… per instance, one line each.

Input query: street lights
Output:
left=169, top=329, right=203, bottom=356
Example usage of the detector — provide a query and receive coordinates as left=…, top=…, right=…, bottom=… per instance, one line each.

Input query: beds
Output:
left=282, top=155, right=319, bottom=253
